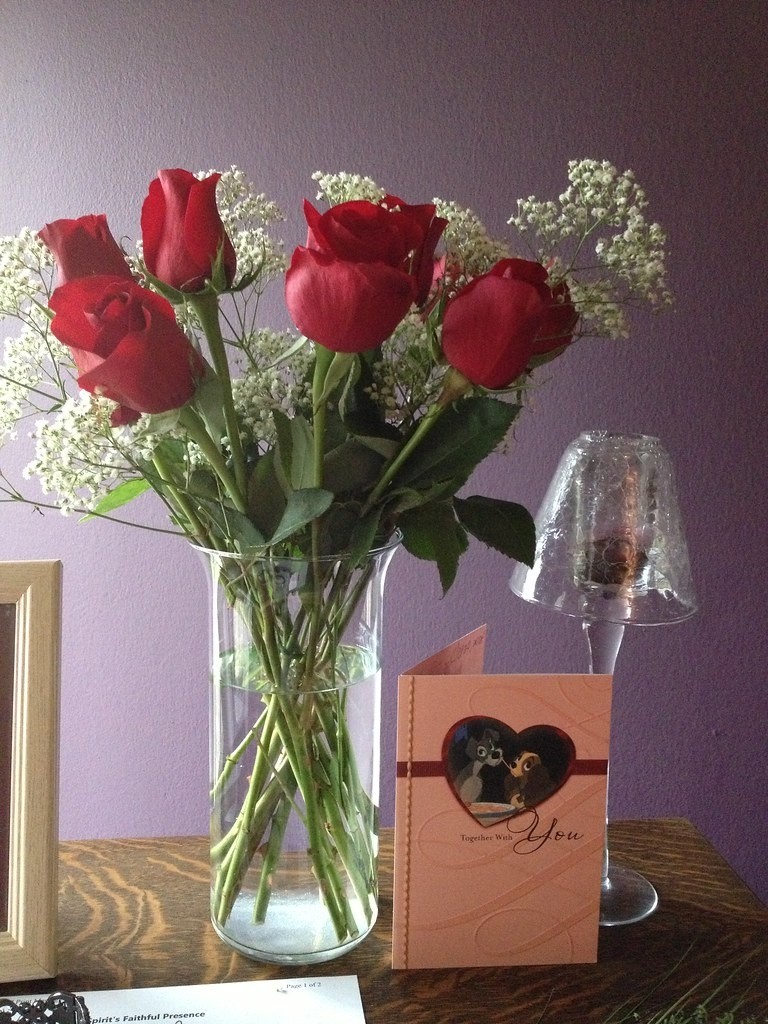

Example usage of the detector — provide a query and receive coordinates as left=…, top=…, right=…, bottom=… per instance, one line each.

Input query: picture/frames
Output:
left=0, top=560, right=63, bottom=986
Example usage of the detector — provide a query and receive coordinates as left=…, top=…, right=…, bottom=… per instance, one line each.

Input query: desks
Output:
left=0, top=814, right=767, bottom=1024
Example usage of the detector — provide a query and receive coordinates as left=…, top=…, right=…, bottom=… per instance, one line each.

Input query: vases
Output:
left=184, top=519, right=410, bottom=969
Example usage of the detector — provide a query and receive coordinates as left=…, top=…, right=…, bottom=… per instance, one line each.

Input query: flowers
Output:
left=0, top=154, right=672, bottom=950
